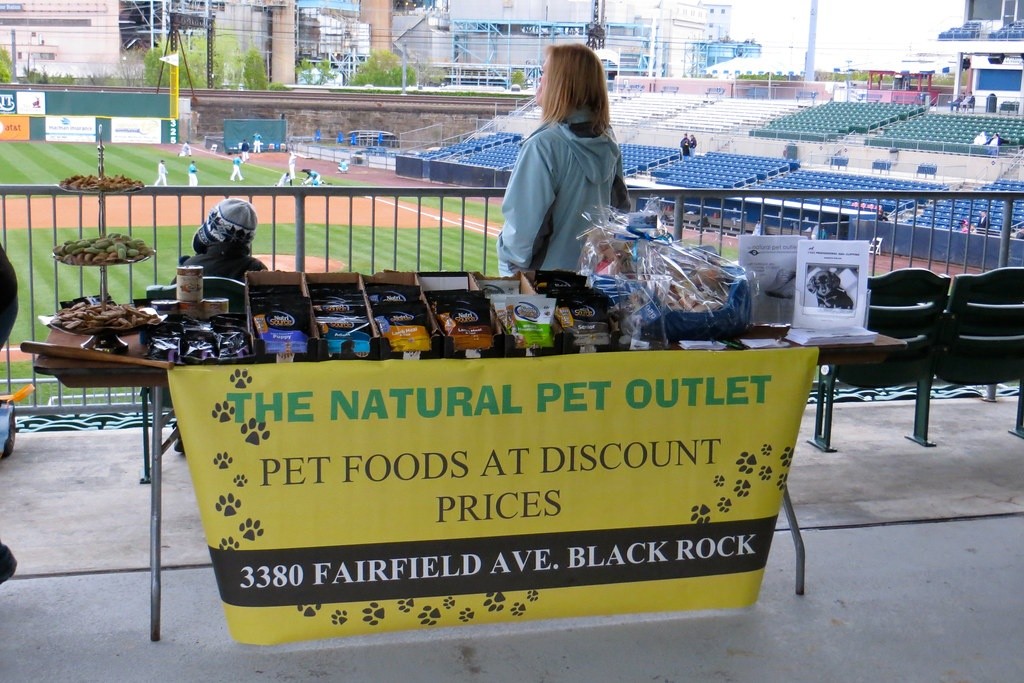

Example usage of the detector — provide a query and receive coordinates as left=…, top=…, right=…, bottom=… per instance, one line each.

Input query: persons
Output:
left=977, top=210, right=990, bottom=229
left=974, top=131, right=1002, bottom=155
left=1016, top=226, right=1024, bottom=239
left=178, top=142, right=191, bottom=156
left=496, top=43, right=630, bottom=278
left=230, top=156, right=243, bottom=181
left=680, top=133, right=697, bottom=160
left=274, top=151, right=325, bottom=186
left=877, top=209, right=888, bottom=221
left=338, top=159, right=348, bottom=173
left=241, top=139, right=249, bottom=161
left=951, top=92, right=975, bottom=112
left=0, top=244, right=18, bottom=587
left=153, top=160, right=169, bottom=185
left=252, top=131, right=262, bottom=153
left=170, top=198, right=268, bottom=287
left=189, top=161, right=198, bottom=186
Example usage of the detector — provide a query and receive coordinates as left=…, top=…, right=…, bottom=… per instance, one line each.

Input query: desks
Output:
left=34, top=327, right=807, bottom=642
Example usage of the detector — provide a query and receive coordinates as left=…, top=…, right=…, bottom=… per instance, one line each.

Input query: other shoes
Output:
left=766, top=269, right=797, bottom=299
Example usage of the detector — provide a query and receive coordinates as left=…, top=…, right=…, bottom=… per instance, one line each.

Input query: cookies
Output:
left=59, top=174, right=143, bottom=191
left=51, top=302, right=160, bottom=330
left=52, top=232, right=152, bottom=263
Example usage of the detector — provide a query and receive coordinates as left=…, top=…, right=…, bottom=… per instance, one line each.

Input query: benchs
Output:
left=746, top=88, right=767, bottom=100
left=661, top=86, right=679, bottom=96
left=705, top=87, right=726, bottom=98
left=858, top=94, right=882, bottom=103
left=796, top=91, right=816, bottom=104
left=891, top=95, right=916, bottom=105
left=951, top=96, right=976, bottom=113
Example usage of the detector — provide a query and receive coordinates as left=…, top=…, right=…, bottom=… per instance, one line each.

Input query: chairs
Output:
left=268, top=143, right=287, bottom=152
left=14, top=411, right=177, bottom=432
left=210, top=144, right=218, bottom=153
left=418, top=101, right=1024, bottom=238
left=938, top=20, right=1024, bottom=38
left=139, top=277, right=246, bottom=483
left=804, top=268, right=1024, bottom=453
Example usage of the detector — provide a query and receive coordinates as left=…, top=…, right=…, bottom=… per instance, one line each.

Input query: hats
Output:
left=193, top=198, right=259, bottom=254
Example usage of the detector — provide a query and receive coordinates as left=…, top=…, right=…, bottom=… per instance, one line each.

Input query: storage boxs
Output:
left=244, top=270, right=621, bottom=363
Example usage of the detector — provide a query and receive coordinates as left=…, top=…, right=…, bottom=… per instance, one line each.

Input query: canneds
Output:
left=151, top=265, right=229, bottom=321
